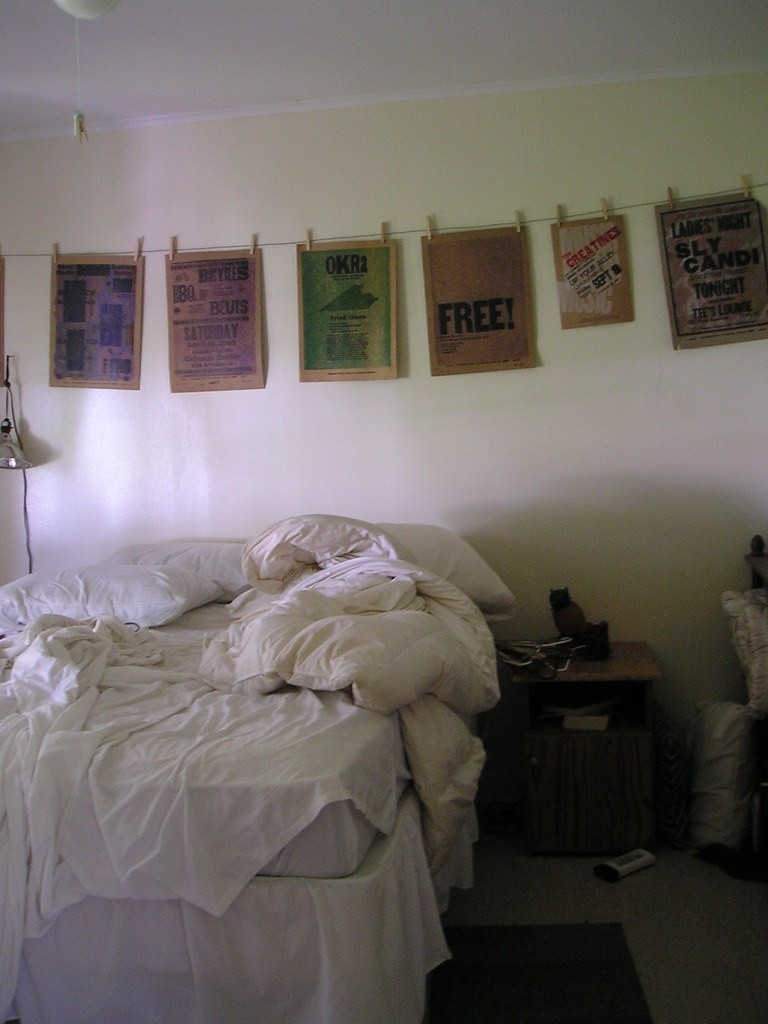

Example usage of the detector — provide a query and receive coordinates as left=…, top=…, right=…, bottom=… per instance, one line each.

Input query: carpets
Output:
left=423, top=921, right=652, bottom=1024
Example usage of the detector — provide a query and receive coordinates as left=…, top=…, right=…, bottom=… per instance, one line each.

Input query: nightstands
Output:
left=511, top=640, right=662, bottom=855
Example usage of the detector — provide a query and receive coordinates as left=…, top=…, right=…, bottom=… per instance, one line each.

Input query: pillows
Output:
left=370, top=522, right=516, bottom=616
left=721, top=587, right=768, bottom=719
left=96, top=542, right=253, bottom=604
left=0, top=565, right=224, bottom=633
left=684, top=701, right=753, bottom=850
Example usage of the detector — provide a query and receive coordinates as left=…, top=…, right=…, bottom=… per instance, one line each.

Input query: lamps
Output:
left=0, top=355, right=34, bottom=470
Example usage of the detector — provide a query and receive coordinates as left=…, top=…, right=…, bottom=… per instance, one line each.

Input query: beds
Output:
left=0, top=569, right=481, bottom=1024
